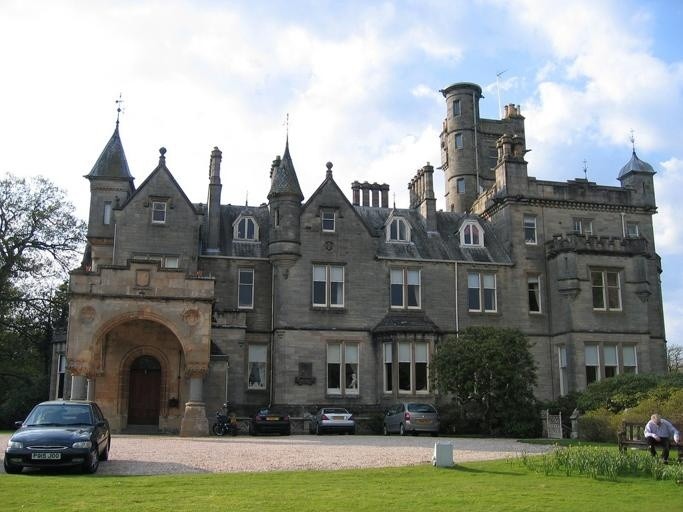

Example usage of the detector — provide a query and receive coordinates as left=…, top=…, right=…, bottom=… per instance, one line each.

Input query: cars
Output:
left=4, top=400, right=110, bottom=474
left=384, top=402, right=440, bottom=435
left=249, top=407, right=356, bottom=435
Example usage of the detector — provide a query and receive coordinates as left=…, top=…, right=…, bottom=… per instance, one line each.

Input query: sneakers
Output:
left=662, top=457, right=668, bottom=463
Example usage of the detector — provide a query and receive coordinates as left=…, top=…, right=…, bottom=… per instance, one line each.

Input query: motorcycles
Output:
left=213, top=404, right=241, bottom=436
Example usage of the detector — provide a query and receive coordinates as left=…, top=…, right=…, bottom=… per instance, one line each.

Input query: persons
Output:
left=644, top=413, right=679, bottom=465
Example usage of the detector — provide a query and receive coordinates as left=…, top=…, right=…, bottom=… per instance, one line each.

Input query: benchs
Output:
left=617, top=420, right=683, bottom=460
